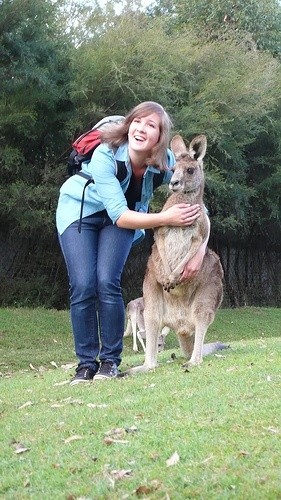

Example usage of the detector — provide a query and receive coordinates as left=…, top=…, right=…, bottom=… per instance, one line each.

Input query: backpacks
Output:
left=67, top=116, right=165, bottom=202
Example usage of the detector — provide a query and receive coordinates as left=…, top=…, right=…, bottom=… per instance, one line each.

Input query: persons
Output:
left=56, top=102, right=211, bottom=384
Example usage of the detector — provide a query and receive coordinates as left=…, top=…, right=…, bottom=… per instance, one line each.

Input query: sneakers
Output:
left=93, top=361, right=122, bottom=380
left=70, top=365, right=99, bottom=386
